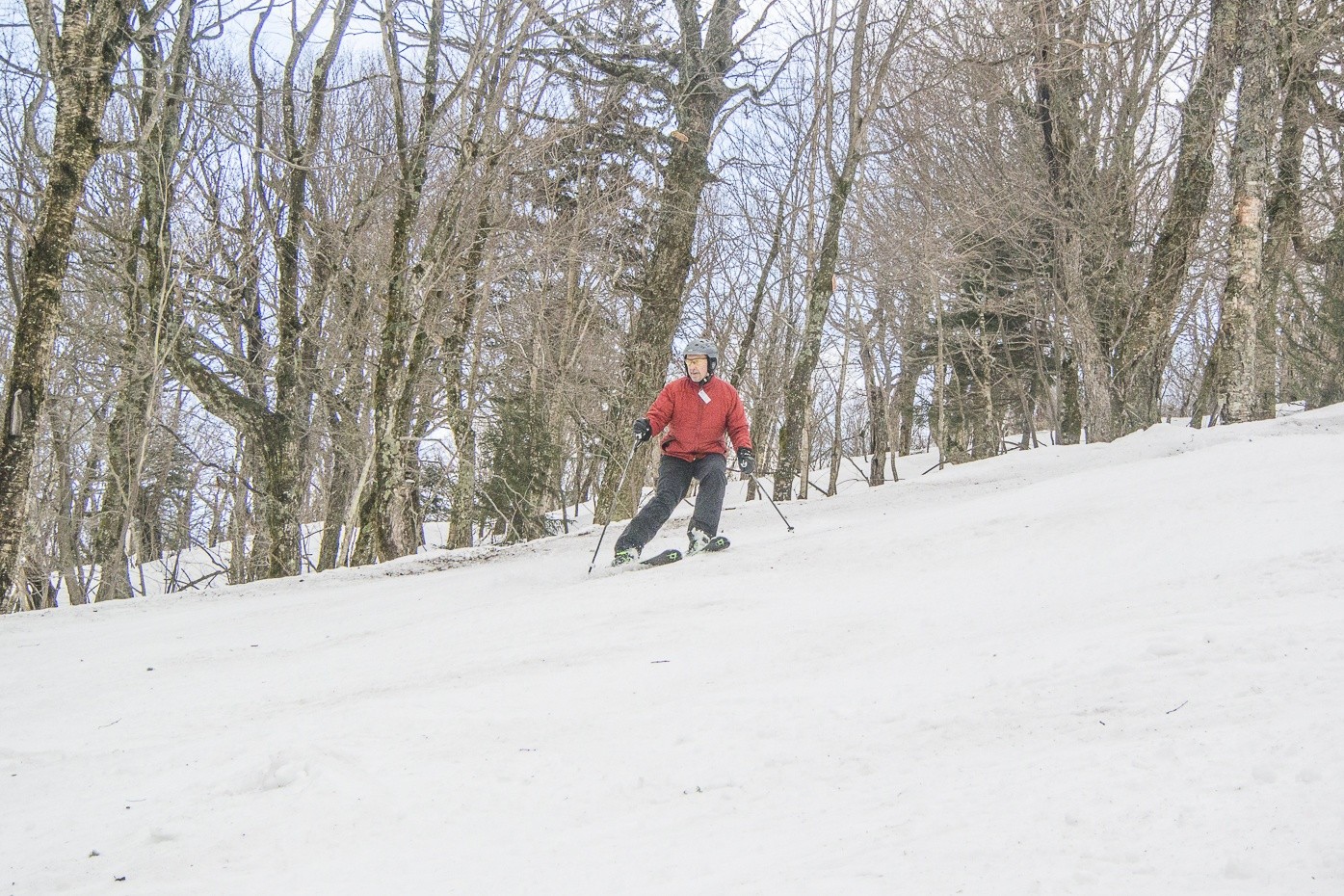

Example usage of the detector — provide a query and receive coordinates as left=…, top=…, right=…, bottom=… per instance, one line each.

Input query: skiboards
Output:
left=601, top=535, right=730, bottom=571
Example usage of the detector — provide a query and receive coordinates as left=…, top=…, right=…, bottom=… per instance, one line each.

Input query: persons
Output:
left=611, top=338, right=755, bottom=565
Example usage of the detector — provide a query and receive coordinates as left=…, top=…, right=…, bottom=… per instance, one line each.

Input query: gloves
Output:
left=632, top=417, right=652, bottom=442
left=737, top=447, right=757, bottom=475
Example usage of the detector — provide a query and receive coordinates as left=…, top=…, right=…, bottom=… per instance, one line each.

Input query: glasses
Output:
left=685, top=357, right=709, bottom=365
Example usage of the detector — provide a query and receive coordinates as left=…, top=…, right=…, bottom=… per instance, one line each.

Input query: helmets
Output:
left=681, top=338, right=718, bottom=358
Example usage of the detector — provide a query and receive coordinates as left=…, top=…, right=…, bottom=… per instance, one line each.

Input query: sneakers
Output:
left=689, top=540, right=710, bottom=552
left=612, top=553, right=635, bottom=566
